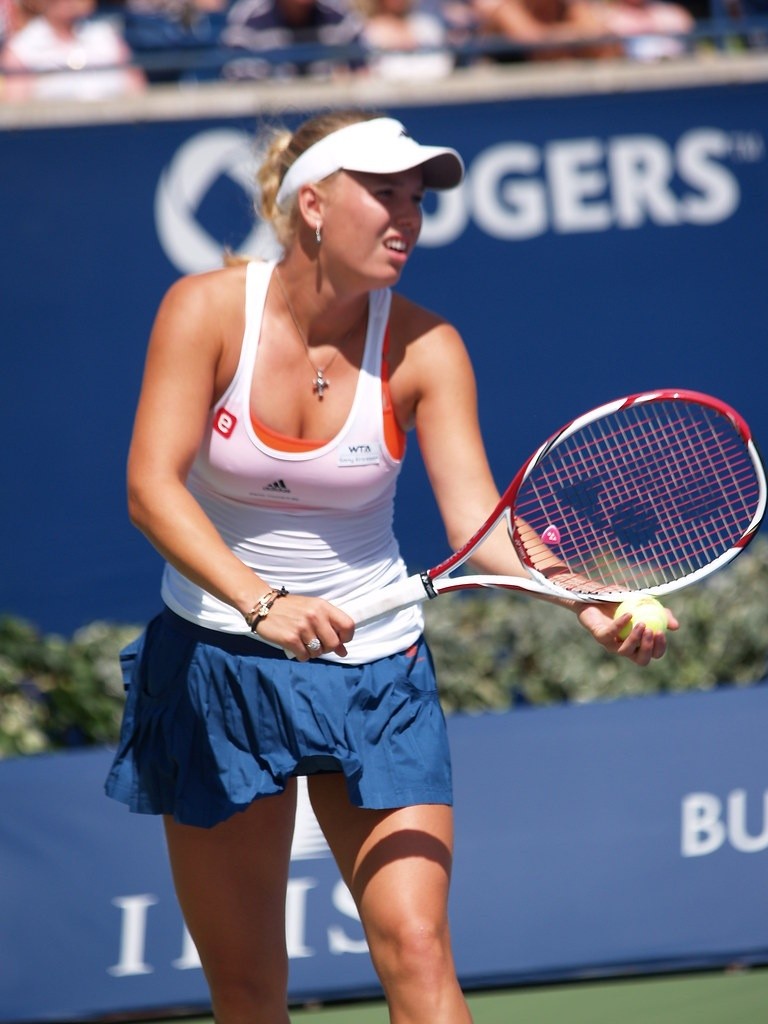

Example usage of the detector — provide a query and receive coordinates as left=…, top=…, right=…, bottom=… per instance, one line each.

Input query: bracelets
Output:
left=539, top=566, right=578, bottom=578
left=245, top=585, right=289, bottom=634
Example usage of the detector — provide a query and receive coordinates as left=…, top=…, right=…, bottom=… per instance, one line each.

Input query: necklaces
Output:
left=274, top=267, right=369, bottom=398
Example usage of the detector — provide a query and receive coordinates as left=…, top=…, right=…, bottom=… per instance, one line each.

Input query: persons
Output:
left=101, top=113, right=682, bottom=1024
left=0, top=0, right=693, bottom=105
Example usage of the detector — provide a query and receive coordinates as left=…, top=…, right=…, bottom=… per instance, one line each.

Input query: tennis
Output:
left=613, top=593, right=668, bottom=643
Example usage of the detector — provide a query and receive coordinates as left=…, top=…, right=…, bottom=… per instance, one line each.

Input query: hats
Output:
left=275, top=116, right=464, bottom=214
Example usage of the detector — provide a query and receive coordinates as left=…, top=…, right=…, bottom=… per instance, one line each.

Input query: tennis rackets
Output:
left=279, top=386, right=768, bottom=661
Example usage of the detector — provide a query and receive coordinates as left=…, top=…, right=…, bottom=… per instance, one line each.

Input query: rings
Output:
left=306, top=639, right=321, bottom=651
